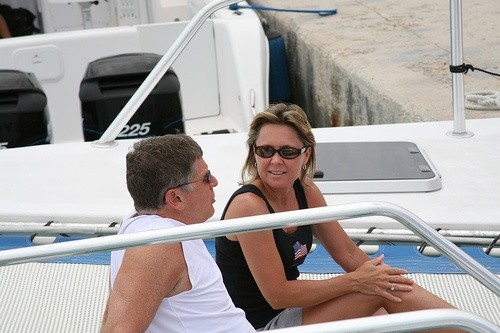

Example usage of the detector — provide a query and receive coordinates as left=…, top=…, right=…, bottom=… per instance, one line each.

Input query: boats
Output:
left=3, top=1, right=500, bottom=332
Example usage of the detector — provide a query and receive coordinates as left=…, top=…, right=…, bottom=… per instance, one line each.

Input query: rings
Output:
left=391, top=282, right=395, bottom=290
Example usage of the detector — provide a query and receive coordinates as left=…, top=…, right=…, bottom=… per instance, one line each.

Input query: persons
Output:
left=216, top=105, right=470, bottom=333
left=101, top=134, right=258, bottom=333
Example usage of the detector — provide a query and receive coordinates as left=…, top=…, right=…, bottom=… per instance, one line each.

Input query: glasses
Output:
left=253, top=143, right=307, bottom=159
left=163, top=170, right=211, bottom=203
